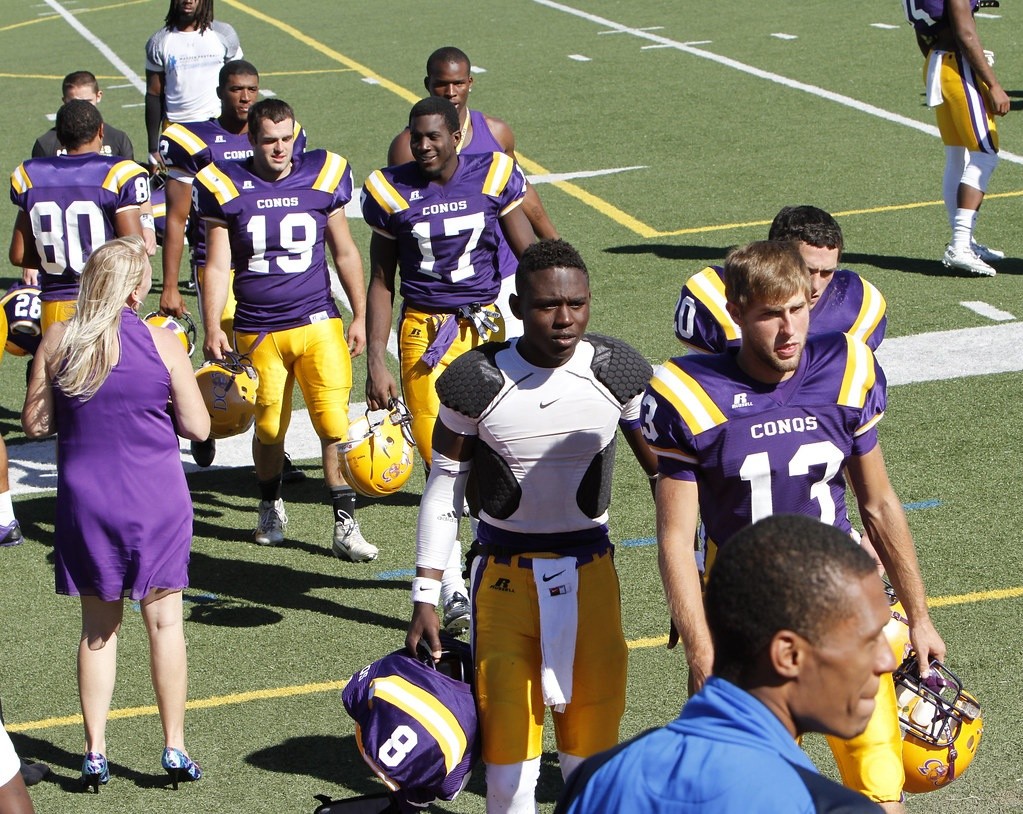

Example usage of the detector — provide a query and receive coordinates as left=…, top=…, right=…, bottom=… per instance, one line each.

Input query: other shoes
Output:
left=282, top=456, right=305, bottom=483
left=0, top=519, right=24, bottom=547
left=191, top=438, right=215, bottom=467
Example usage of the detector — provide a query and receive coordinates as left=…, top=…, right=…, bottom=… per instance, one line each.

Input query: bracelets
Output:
left=412, top=577, right=443, bottom=607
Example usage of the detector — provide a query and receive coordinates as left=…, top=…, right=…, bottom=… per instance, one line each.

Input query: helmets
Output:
left=882, top=589, right=911, bottom=667
left=193, top=351, right=259, bottom=439
left=337, top=399, right=417, bottom=499
left=135, top=160, right=168, bottom=192
left=144, top=311, right=197, bottom=357
left=892, top=652, right=983, bottom=793
left=0, top=285, right=43, bottom=357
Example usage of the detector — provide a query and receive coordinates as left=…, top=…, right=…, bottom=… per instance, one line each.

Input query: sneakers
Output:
left=255, top=498, right=286, bottom=545
left=332, top=519, right=379, bottom=562
left=972, top=244, right=1003, bottom=261
left=443, top=598, right=472, bottom=634
left=941, top=246, right=996, bottom=276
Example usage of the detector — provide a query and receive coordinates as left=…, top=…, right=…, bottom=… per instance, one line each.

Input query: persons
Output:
left=0, top=0, right=559, bottom=814
left=672, top=205, right=891, bottom=349
left=905, top=0, right=1010, bottom=278
left=24, top=237, right=212, bottom=794
left=554, top=515, right=898, bottom=814
left=638, top=239, right=949, bottom=812
left=405, top=237, right=656, bottom=812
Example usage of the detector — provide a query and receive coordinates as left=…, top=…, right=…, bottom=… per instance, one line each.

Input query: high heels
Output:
left=161, top=747, right=201, bottom=790
left=82, top=752, right=109, bottom=793
